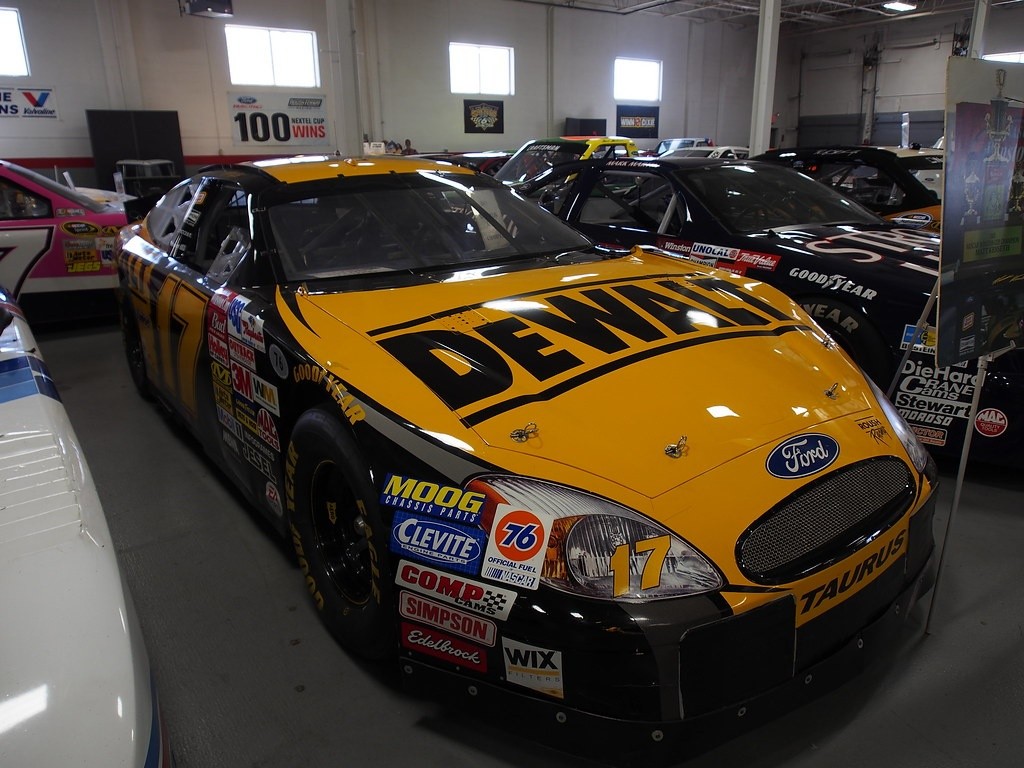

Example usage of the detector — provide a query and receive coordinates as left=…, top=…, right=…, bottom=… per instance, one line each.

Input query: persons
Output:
left=400, top=139, right=418, bottom=155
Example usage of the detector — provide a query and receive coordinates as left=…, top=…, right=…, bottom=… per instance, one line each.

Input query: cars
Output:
left=0, top=283, right=177, bottom=768
left=750, top=146, right=943, bottom=233
left=0, top=158, right=144, bottom=325
left=486, top=157, right=1024, bottom=472
left=403, top=135, right=750, bottom=198
left=116, top=152, right=943, bottom=768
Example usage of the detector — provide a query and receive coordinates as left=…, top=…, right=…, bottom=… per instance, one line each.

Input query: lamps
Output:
left=179, top=0, right=233, bottom=19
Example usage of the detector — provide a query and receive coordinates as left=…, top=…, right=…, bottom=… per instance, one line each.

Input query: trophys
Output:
left=959, top=68, right=1024, bottom=226
